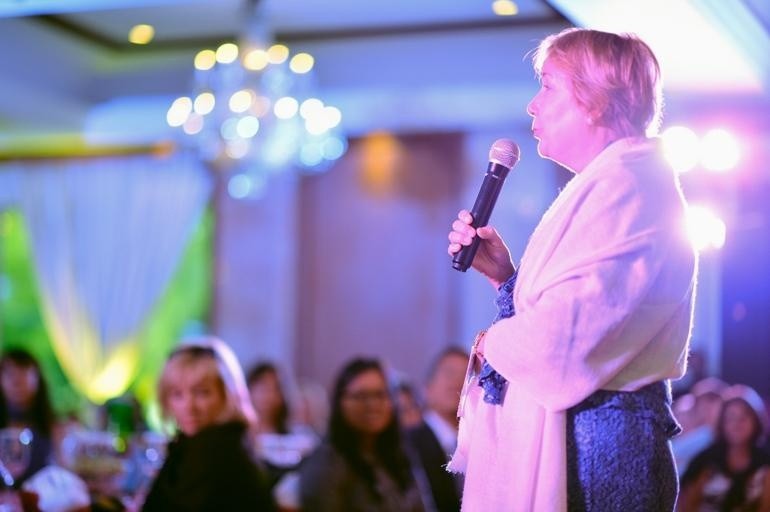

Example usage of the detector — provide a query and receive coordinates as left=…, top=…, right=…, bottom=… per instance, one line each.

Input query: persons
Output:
left=407, top=348, right=476, bottom=512
left=669, top=379, right=721, bottom=480
left=447, top=27, right=698, bottom=512
left=297, top=357, right=420, bottom=511
left=100, top=396, right=168, bottom=510
left=137, top=342, right=279, bottom=512
left=675, top=388, right=766, bottom=512
left=1, top=348, right=79, bottom=512
left=396, top=385, right=419, bottom=428
left=248, top=362, right=316, bottom=466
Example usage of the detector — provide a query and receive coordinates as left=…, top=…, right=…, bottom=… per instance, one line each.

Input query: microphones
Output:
left=452, top=138, right=520, bottom=273
